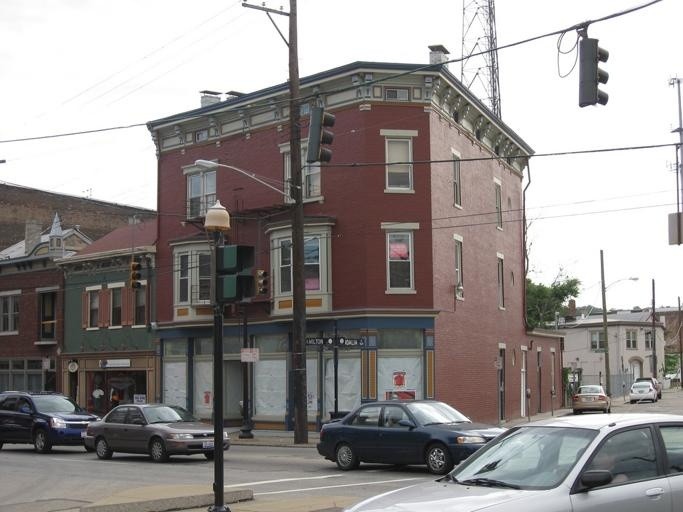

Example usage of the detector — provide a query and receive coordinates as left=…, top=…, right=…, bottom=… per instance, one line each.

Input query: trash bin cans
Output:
left=94, top=395, right=101, bottom=409
left=327, top=411, right=350, bottom=420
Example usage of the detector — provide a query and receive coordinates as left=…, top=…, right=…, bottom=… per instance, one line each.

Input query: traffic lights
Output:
left=579, top=38, right=609, bottom=107
left=129, top=262, right=142, bottom=290
left=306, top=106, right=335, bottom=162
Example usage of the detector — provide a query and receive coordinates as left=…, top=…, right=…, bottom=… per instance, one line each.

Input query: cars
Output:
left=0, top=391, right=230, bottom=460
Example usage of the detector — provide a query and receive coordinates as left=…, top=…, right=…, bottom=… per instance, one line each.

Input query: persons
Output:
left=591, top=442, right=630, bottom=484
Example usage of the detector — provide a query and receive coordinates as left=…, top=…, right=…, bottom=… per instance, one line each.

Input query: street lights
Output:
left=600, top=249, right=638, bottom=397
left=195, top=0, right=309, bottom=445
left=203, top=198, right=232, bottom=512
